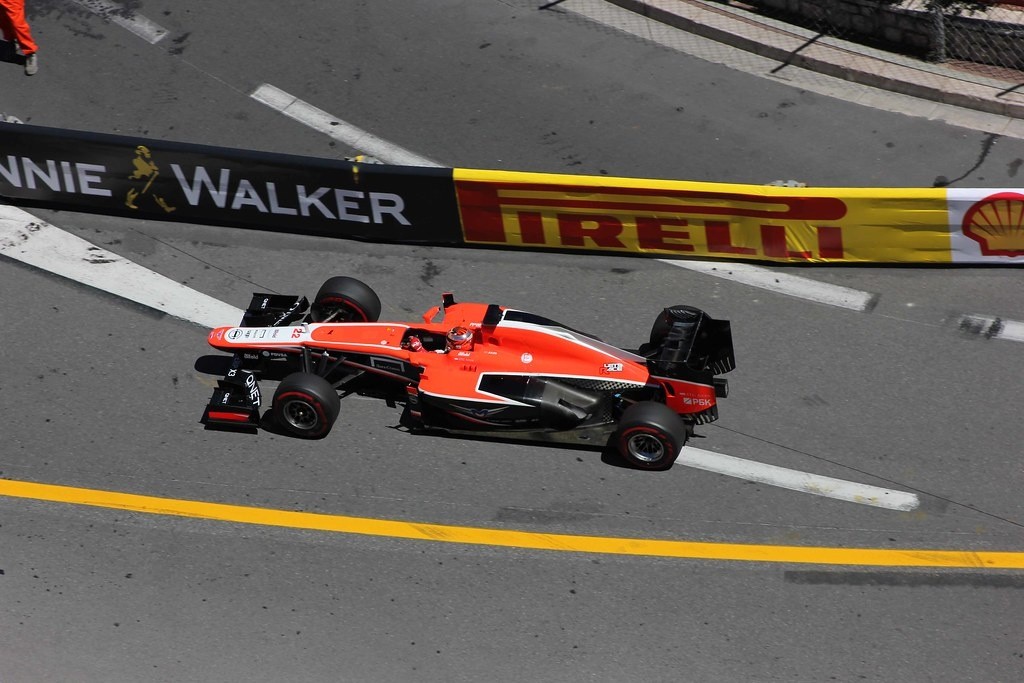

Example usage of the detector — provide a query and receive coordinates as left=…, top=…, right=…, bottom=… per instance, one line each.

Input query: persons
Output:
left=408, top=326, right=472, bottom=355
left=0, top=0, right=39, bottom=75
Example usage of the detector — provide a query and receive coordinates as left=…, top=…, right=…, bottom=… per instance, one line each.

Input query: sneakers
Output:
left=25, top=51, right=38, bottom=75
left=4, top=38, right=19, bottom=51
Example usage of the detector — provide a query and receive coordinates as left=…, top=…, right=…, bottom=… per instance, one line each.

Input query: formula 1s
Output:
left=204, top=276, right=736, bottom=470
